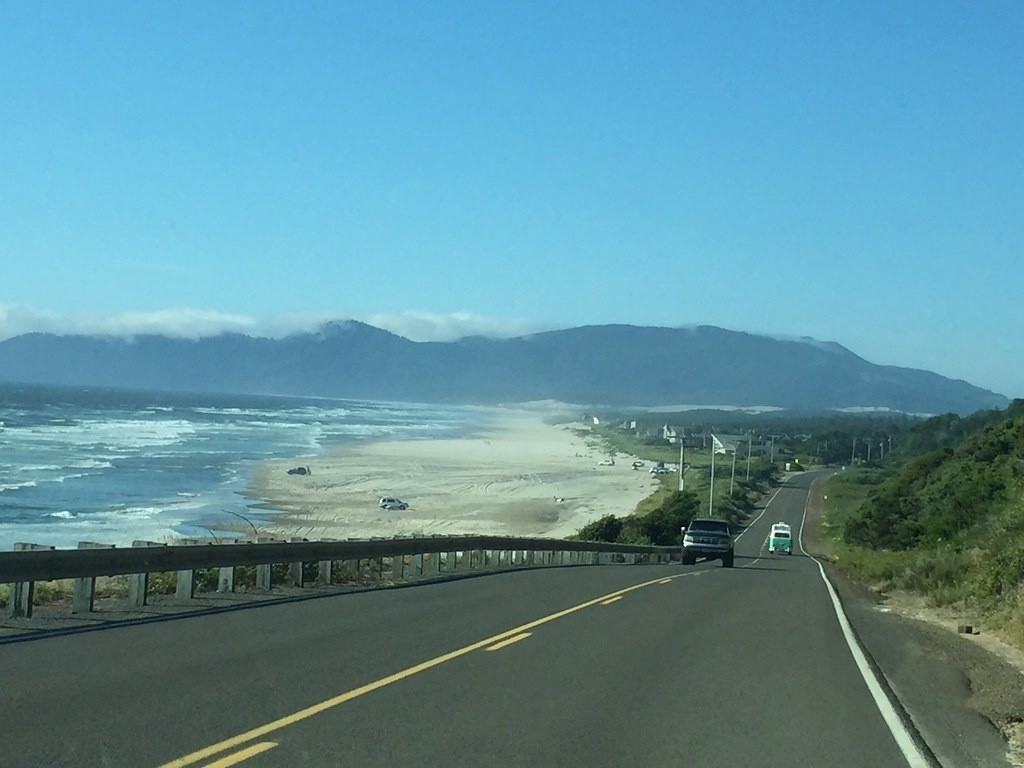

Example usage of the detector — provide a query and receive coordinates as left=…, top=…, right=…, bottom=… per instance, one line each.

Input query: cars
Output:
left=598, top=460, right=616, bottom=466
left=632, top=457, right=678, bottom=475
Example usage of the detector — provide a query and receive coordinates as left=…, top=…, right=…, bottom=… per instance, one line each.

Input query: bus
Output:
left=767, top=523, right=794, bottom=555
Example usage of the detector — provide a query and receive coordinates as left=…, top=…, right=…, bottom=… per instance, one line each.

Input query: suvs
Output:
left=287, top=466, right=307, bottom=476
left=680, top=519, right=736, bottom=567
left=380, top=495, right=410, bottom=511
